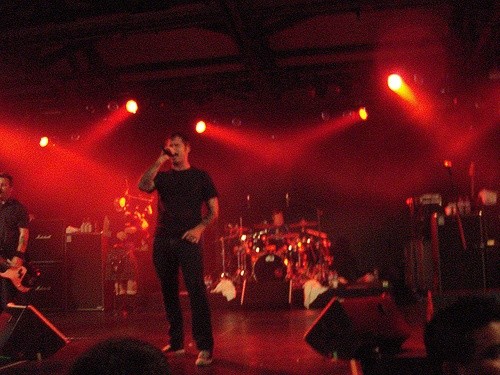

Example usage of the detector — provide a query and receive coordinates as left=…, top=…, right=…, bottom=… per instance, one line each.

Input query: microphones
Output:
left=163, top=148, right=178, bottom=159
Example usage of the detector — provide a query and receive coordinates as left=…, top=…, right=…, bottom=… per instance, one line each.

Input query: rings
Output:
left=193, top=239, right=196, bottom=240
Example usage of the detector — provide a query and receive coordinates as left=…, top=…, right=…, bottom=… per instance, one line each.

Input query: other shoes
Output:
left=162, top=344, right=185, bottom=355
left=195, top=350, right=213, bottom=366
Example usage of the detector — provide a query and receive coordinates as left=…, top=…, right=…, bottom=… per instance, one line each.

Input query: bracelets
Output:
left=14, top=250, right=24, bottom=258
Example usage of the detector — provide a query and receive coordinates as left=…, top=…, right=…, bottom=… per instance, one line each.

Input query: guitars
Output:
left=0, top=255, right=39, bottom=292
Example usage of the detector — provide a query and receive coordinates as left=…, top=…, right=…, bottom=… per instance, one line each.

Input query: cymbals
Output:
left=256, top=222, right=317, bottom=229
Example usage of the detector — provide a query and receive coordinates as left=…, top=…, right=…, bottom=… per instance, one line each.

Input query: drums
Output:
left=239, top=235, right=332, bottom=266
left=253, top=253, right=288, bottom=281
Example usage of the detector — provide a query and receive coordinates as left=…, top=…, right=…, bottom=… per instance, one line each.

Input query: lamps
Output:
left=113, top=197, right=126, bottom=212
left=134, top=204, right=152, bottom=214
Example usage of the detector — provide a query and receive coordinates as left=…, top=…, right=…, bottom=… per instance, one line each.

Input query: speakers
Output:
left=28, top=262, right=64, bottom=312
left=436, top=212, right=488, bottom=295
left=303, top=294, right=411, bottom=358
left=28, top=219, right=63, bottom=262
left=64, top=234, right=105, bottom=311
left=0, top=301, right=68, bottom=361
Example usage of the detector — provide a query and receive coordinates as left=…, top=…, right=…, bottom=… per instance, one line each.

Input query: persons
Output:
left=138, top=132, right=219, bottom=366
left=423, top=296, right=500, bottom=375
left=0, top=173, right=30, bottom=316
left=70, top=338, right=170, bottom=375
